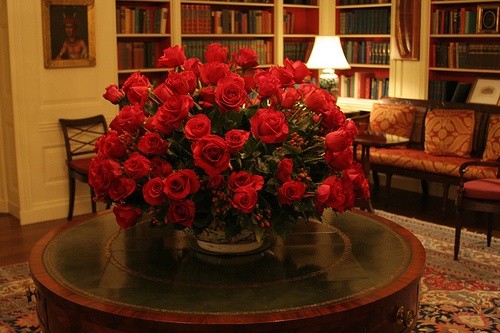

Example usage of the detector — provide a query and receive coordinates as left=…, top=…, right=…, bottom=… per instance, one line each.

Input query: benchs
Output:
left=345, top=96, right=500, bottom=221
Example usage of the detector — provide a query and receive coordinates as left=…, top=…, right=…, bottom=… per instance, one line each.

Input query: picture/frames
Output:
left=476, top=3, right=500, bottom=34
left=40, top=0, right=97, bottom=69
left=465, top=76, right=500, bottom=103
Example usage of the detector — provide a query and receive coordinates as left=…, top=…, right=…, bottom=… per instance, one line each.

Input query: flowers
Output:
left=86, top=45, right=371, bottom=243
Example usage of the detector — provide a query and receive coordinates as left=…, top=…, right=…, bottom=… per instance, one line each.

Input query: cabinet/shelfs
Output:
left=115, top=0, right=500, bottom=103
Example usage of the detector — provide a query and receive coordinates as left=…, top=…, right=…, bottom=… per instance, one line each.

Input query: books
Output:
left=340, top=72, right=389, bottom=100
left=284, top=0, right=317, bottom=5
left=342, top=0, right=390, bottom=5
left=433, top=9, right=476, bottom=34
left=283, top=12, right=294, bottom=34
left=284, top=42, right=313, bottom=62
left=433, top=42, right=500, bottom=70
left=181, top=0, right=272, bottom=34
left=340, top=8, right=391, bottom=34
left=118, top=42, right=160, bottom=69
left=430, top=80, right=472, bottom=103
left=342, top=41, right=390, bottom=64
left=182, top=39, right=273, bottom=65
left=116, top=7, right=168, bottom=33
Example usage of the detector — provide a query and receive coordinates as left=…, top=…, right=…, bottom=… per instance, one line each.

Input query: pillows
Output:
left=367, top=103, right=416, bottom=150
left=424, top=110, right=474, bottom=159
left=480, top=115, right=500, bottom=162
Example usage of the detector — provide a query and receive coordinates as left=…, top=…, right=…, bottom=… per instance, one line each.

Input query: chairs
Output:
left=453, top=158, right=500, bottom=261
left=58, top=114, right=112, bottom=222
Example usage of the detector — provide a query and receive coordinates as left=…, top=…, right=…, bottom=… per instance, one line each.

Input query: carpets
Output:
left=0, top=205, right=500, bottom=333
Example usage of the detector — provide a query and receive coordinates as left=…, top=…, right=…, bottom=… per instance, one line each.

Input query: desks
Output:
left=335, top=97, right=381, bottom=117
left=29, top=208, right=426, bottom=333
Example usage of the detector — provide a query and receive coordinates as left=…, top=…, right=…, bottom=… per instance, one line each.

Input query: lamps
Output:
left=305, top=36, right=351, bottom=104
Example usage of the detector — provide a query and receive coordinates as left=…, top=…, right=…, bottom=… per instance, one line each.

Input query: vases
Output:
left=196, top=218, right=264, bottom=253
